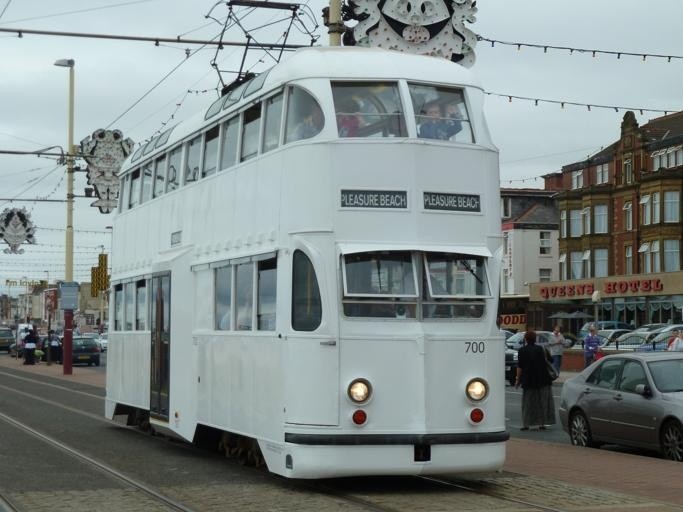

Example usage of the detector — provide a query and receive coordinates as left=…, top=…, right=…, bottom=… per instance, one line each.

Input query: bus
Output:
left=102, top=42, right=517, bottom=490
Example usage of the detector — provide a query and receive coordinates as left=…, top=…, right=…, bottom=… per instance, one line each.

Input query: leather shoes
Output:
left=520, top=426, right=546, bottom=432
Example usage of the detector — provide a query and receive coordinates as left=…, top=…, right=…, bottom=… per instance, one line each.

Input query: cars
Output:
left=555, top=351, right=682, bottom=465
left=0, top=321, right=107, bottom=368
left=496, top=320, right=682, bottom=385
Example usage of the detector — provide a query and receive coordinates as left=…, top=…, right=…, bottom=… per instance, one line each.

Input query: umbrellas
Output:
left=547, top=312, right=569, bottom=320
left=571, top=312, right=593, bottom=340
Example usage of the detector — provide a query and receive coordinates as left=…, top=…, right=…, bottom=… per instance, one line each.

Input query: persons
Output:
left=665, top=329, right=683, bottom=351
left=582, top=328, right=601, bottom=368
left=423, top=104, right=471, bottom=141
left=18, top=326, right=63, bottom=365
left=549, top=326, right=567, bottom=378
left=513, top=329, right=555, bottom=431
left=294, top=102, right=405, bottom=140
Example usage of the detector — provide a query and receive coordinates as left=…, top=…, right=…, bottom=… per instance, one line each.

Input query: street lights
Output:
left=42, top=269, right=50, bottom=297
left=22, top=275, right=29, bottom=315
left=52, top=54, right=78, bottom=376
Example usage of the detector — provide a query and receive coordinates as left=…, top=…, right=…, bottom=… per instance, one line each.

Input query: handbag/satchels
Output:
left=541, top=345, right=559, bottom=382
